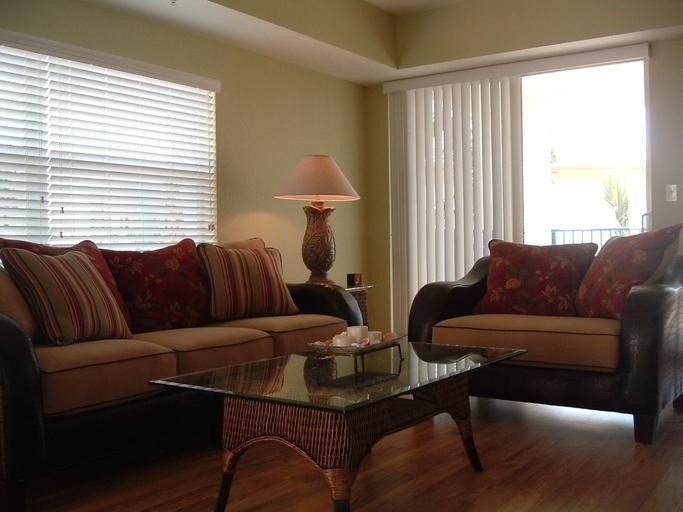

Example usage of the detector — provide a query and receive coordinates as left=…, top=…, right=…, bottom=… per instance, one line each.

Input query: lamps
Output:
left=274, top=154, right=360, bottom=286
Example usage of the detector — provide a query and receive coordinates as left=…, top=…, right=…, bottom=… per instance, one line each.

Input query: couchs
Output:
left=406, top=255, right=682, bottom=444
left=0, top=238, right=364, bottom=511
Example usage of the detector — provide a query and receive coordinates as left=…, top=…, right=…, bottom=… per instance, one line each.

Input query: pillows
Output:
left=0, top=239, right=298, bottom=346
left=472, top=224, right=682, bottom=319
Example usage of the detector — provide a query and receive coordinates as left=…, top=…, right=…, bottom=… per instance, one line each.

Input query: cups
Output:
left=334, top=324, right=382, bottom=348
